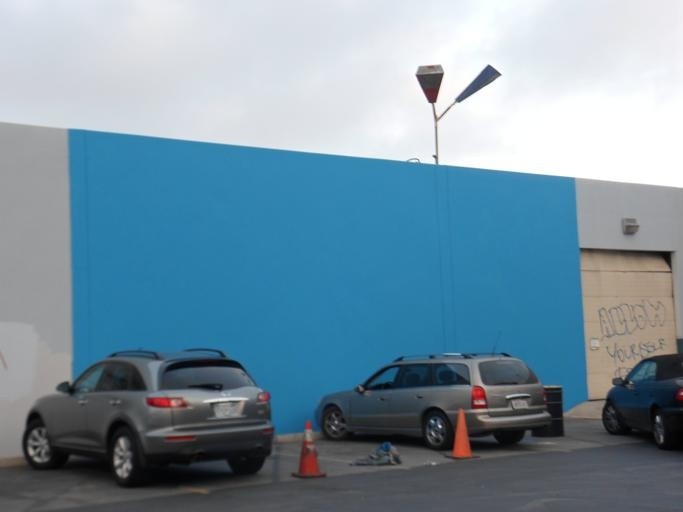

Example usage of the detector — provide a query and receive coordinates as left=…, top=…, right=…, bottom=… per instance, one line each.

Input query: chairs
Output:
left=404, top=369, right=456, bottom=385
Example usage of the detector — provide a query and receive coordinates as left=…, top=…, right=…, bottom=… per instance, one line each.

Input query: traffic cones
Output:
left=444, top=409, right=478, bottom=459
left=292, top=421, right=325, bottom=478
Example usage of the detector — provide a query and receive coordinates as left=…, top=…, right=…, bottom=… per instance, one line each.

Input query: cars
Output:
left=602, top=354, right=683, bottom=450
left=315, top=353, right=551, bottom=450
left=23, top=348, right=274, bottom=487
